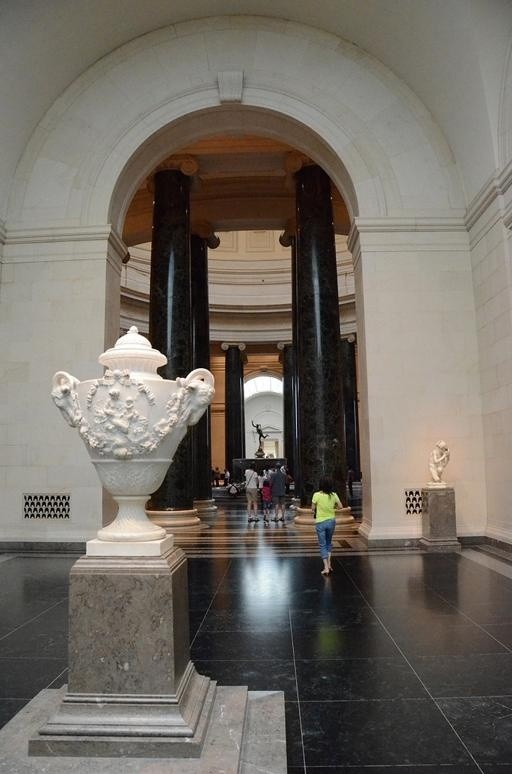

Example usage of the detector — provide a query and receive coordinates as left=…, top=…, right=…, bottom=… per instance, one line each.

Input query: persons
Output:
left=429, top=440, right=451, bottom=484
left=213, top=462, right=294, bottom=523
left=308, top=476, right=344, bottom=575
left=252, top=420, right=270, bottom=449
left=347, top=464, right=355, bottom=500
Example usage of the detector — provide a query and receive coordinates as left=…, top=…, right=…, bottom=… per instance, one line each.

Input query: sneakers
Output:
left=248, top=514, right=284, bottom=523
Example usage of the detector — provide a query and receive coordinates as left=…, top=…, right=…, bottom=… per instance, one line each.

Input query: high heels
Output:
left=321, top=568, right=333, bottom=576
